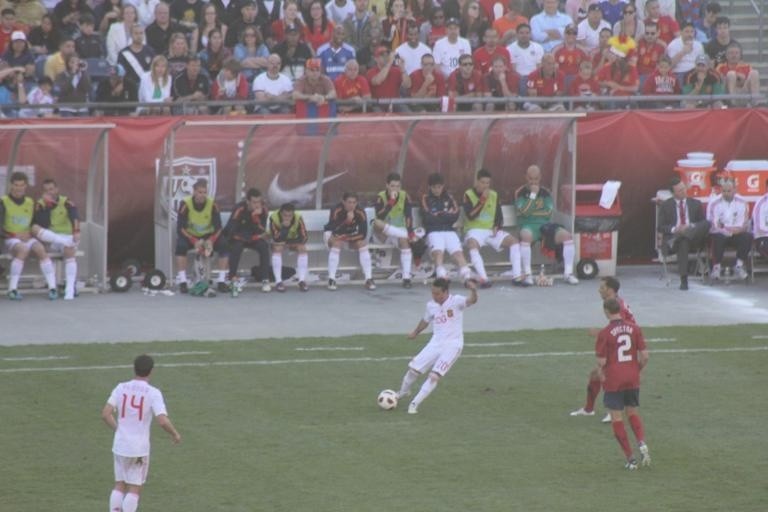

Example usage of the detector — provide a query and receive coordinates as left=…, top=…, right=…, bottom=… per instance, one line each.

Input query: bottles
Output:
left=539, top=264, right=545, bottom=278
left=724, top=267, right=731, bottom=286
left=93, top=274, right=99, bottom=294
left=232, top=268, right=246, bottom=297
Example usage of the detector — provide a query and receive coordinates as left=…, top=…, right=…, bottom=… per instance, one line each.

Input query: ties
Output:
left=679, top=201, right=686, bottom=225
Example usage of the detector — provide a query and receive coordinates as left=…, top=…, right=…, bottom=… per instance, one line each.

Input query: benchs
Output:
left=187, top=203, right=564, bottom=291
left=0, top=247, right=85, bottom=294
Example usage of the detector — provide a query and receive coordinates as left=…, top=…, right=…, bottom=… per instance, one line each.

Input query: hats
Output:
left=10, top=30, right=26, bottom=41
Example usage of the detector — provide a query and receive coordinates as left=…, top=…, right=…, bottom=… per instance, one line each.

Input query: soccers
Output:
left=378, top=390, right=397, bottom=409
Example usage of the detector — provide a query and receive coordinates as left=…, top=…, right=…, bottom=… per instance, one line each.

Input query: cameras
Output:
left=78, top=60, right=86, bottom=68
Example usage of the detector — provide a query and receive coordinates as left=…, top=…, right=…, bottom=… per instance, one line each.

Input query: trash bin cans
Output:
left=571, top=183, right=623, bottom=279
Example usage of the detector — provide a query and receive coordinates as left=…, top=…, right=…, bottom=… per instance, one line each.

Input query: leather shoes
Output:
left=680, top=277, right=688, bottom=290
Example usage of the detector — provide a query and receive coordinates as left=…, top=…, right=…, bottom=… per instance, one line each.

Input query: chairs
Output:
left=653, top=215, right=767, bottom=292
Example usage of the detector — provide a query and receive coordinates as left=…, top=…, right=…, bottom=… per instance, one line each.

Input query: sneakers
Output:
left=397, top=391, right=411, bottom=399
left=570, top=408, right=595, bottom=416
left=602, top=413, right=611, bottom=422
left=710, top=267, right=720, bottom=280
left=736, top=267, right=748, bottom=280
left=638, top=441, right=650, bottom=466
left=8, top=235, right=75, bottom=300
left=408, top=403, right=417, bottom=414
left=624, top=458, right=638, bottom=471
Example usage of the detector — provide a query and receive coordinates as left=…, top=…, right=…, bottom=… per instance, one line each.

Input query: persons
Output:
left=596, top=297, right=652, bottom=472
left=655, top=175, right=768, bottom=290
left=103, top=355, right=182, bottom=512
left=2, top=1, right=759, bottom=117
left=178, top=163, right=580, bottom=297
left=398, top=278, right=477, bottom=413
left=2, top=171, right=82, bottom=299
left=570, top=275, right=636, bottom=422
